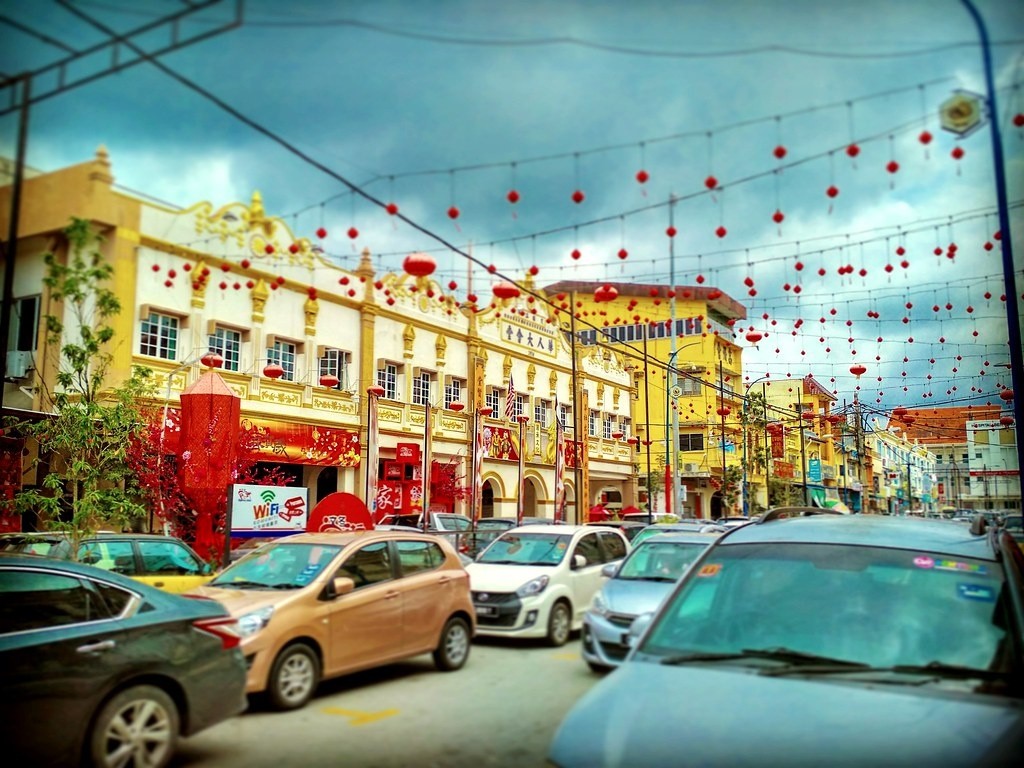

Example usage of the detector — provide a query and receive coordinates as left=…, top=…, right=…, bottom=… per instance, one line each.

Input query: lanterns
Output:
left=709, top=476, right=730, bottom=493
left=178, top=364, right=241, bottom=516
left=145, top=100, right=1024, bottom=452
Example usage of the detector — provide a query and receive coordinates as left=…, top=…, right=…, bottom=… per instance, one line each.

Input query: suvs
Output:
left=1, top=530, right=220, bottom=623
left=544, top=504, right=1024, bottom=768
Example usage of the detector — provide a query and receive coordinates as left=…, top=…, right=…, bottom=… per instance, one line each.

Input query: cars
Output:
left=581, top=532, right=726, bottom=674
left=376, top=509, right=568, bottom=567
left=585, top=511, right=760, bottom=541
left=463, top=524, right=632, bottom=648
left=180, top=529, right=477, bottom=711
left=0, top=555, right=250, bottom=767
left=630, top=523, right=731, bottom=572
left=952, top=508, right=1024, bottom=544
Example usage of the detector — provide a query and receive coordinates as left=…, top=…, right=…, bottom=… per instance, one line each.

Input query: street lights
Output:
left=665, top=342, right=702, bottom=512
left=743, top=376, right=769, bottom=516
left=822, top=427, right=901, bottom=513
